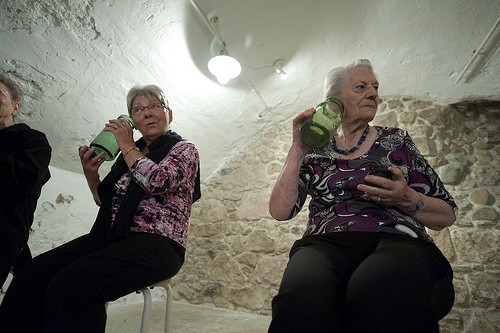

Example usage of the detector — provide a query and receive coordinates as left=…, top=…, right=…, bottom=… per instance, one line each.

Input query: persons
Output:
left=267, top=57, right=459, bottom=332
left=10, top=84, right=202, bottom=333
left=0, top=72, right=51, bottom=301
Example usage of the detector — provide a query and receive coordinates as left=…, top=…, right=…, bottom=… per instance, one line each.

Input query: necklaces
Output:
left=331, top=124, right=369, bottom=155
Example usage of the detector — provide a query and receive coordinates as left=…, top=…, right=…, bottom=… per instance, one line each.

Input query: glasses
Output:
left=132, top=103, right=163, bottom=115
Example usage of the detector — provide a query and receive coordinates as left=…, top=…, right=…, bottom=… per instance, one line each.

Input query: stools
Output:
left=136, top=279, right=172, bottom=333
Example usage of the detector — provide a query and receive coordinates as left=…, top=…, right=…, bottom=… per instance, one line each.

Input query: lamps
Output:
left=208, top=46, right=242, bottom=86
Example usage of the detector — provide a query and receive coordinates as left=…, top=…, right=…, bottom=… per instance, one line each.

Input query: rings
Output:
left=376, top=196, right=381, bottom=202
left=113, top=125, right=118, bottom=129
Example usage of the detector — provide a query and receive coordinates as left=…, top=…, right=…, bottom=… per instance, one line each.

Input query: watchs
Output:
left=409, top=192, right=425, bottom=216
left=123, top=146, right=139, bottom=159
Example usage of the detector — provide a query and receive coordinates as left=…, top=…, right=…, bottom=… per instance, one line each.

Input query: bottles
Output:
left=89, top=114, right=136, bottom=161
left=298, top=98, right=344, bottom=149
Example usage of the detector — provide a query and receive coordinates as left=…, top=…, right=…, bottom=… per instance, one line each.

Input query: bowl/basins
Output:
left=364, top=167, right=395, bottom=191
